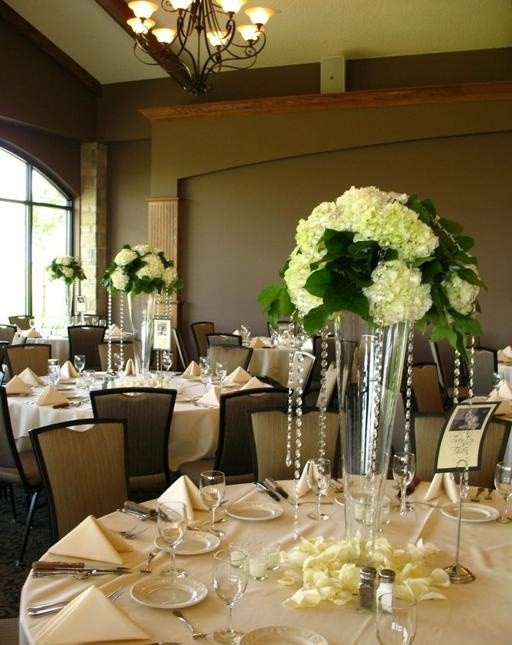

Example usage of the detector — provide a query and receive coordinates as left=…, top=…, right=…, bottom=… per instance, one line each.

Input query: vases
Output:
left=126, top=286, right=160, bottom=386
left=62, top=282, right=72, bottom=337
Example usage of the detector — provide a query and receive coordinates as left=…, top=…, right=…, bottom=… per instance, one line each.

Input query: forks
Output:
left=140, top=548, right=161, bottom=573
left=108, top=525, right=149, bottom=541
left=173, top=610, right=210, bottom=640
left=28, top=588, right=124, bottom=616
left=114, top=506, right=154, bottom=521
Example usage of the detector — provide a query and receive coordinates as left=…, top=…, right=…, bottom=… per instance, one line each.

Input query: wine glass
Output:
left=198, top=470, right=226, bottom=539
left=237, top=320, right=333, bottom=351
left=47, top=349, right=230, bottom=396
left=25, top=314, right=108, bottom=338
left=492, top=369, right=506, bottom=402
left=211, top=547, right=250, bottom=644
left=391, top=453, right=416, bottom=525
left=157, top=502, right=187, bottom=578
left=306, top=458, right=332, bottom=521
left=494, top=462, right=512, bottom=525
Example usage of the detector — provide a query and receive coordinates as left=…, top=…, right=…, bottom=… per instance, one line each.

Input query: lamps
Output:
left=123, top=0, right=277, bottom=98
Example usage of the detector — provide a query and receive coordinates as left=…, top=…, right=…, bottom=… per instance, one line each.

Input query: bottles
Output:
left=357, top=566, right=376, bottom=612
left=376, top=569, right=396, bottom=614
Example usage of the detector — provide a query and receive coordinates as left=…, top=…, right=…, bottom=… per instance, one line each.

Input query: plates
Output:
left=224, top=501, right=284, bottom=522
left=439, top=503, right=501, bottom=523
left=334, top=489, right=391, bottom=509
left=240, top=625, right=328, bottom=645
left=154, top=528, right=220, bottom=555
left=131, top=575, right=209, bottom=609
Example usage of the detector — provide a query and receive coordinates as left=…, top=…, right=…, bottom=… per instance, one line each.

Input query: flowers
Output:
left=253, top=182, right=490, bottom=367
left=99, top=243, right=186, bottom=297
left=46, top=254, right=88, bottom=288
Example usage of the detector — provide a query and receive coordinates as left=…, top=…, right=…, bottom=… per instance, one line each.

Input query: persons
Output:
left=469, top=415, right=481, bottom=430
left=454, top=408, right=478, bottom=430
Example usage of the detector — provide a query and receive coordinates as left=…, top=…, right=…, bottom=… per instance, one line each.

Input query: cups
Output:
left=373, top=593, right=419, bottom=645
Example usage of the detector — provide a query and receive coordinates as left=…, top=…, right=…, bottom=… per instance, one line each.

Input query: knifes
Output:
left=257, top=481, right=282, bottom=502
left=32, top=558, right=132, bottom=579
left=266, top=478, right=289, bottom=499
